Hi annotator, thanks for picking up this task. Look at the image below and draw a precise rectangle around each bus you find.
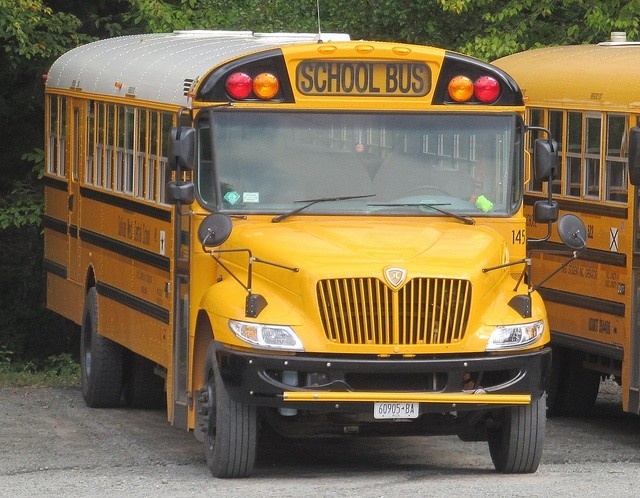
[489,30,640,418]
[44,1,589,475]
